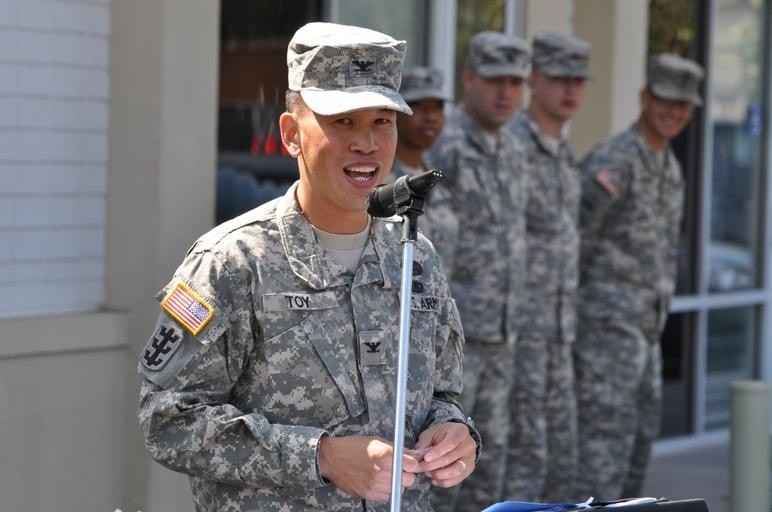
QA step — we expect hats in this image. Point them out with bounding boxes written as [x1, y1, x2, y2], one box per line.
[399, 66, 449, 102]
[468, 30, 594, 77]
[647, 52, 706, 106]
[286, 22, 413, 115]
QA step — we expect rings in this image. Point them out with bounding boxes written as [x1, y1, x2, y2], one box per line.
[455, 459, 467, 470]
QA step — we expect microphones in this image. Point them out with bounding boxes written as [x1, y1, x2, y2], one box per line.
[363, 168, 446, 218]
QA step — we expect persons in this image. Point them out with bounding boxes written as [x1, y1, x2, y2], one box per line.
[135, 19, 482, 512]
[428, 30, 534, 512]
[385, 63, 463, 285]
[576, 51, 708, 503]
[499, 29, 593, 505]
